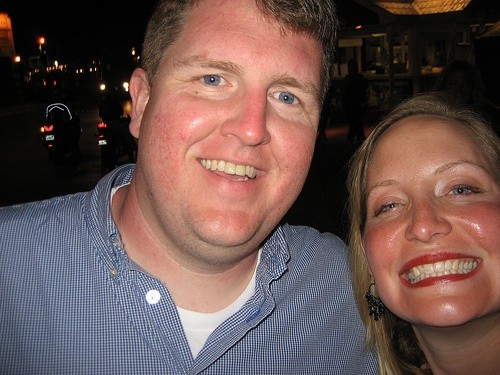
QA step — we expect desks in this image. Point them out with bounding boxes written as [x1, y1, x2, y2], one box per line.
[367, 68, 444, 103]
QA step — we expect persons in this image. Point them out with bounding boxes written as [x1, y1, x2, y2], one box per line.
[0, 0, 383, 375]
[315, 58, 473, 146]
[345, 92, 500, 373]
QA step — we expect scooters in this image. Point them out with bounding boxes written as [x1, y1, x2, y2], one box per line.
[97, 114, 137, 167]
[36, 108, 82, 162]
